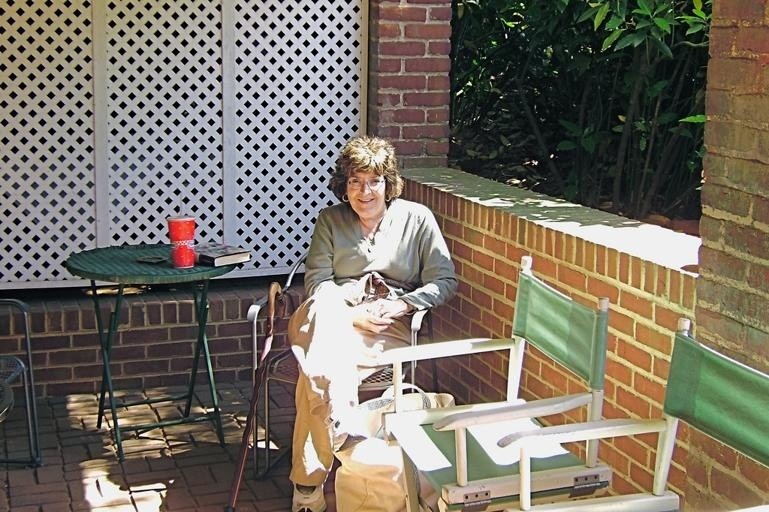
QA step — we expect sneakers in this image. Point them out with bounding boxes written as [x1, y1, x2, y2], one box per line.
[291, 483, 327, 512]
[323, 406, 370, 452]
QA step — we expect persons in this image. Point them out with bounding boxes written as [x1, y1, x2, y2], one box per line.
[287, 134, 459, 511]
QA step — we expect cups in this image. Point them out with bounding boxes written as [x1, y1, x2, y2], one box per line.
[166, 215, 197, 270]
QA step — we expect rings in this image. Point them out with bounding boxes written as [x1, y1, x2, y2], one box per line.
[381, 312, 385, 315]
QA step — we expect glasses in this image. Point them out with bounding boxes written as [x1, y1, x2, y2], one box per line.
[345, 177, 386, 191]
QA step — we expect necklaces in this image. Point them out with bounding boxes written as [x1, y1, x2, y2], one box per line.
[359, 217, 383, 240]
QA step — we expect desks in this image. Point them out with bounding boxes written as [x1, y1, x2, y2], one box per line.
[65, 243, 240, 463]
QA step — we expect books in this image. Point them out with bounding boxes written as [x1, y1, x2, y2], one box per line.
[194, 241, 252, 268]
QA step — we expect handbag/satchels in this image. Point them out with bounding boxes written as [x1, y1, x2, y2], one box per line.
[335, 382, 455, 512]
